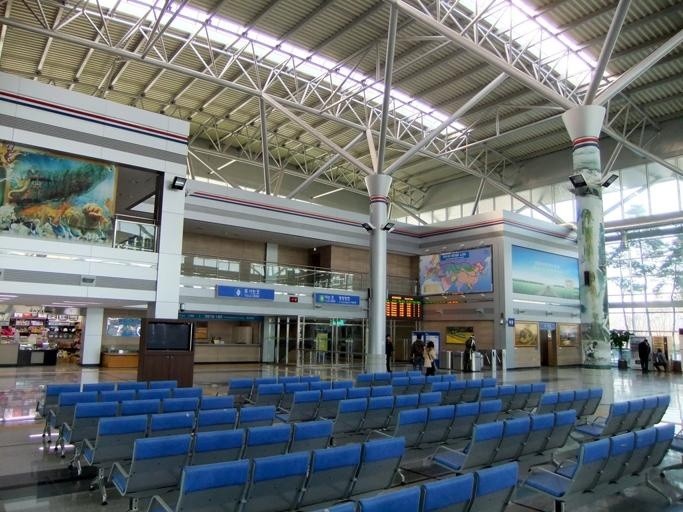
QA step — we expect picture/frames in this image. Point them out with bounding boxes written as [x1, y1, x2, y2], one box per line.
[558, 324, 578, 347]
[511, 244, 581, 301]
[3, 145, 118, 246]
[514, 322, 538, 347]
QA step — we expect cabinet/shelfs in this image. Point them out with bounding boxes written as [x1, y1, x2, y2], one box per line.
[232, 326, 253, 344]
[0, 317, 82, 363]
[136, 318, 195, 388]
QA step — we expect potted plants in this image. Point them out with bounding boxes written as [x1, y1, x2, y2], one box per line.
[609, 329, 629, 369]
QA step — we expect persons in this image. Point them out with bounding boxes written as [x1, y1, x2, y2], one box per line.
[386, 335, 393, 372]
[653, 348, 668, 373]
[638, 340, 650, 373]
[411, 334, 425, 370]
[422, 341, 437, 375]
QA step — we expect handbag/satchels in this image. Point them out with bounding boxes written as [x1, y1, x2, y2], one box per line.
[431, 361, 437, 372]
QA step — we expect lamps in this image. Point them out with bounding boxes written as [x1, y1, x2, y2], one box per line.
[361, 221, 376, 232]
[599, 172, 619, 188]
[568, 172, 587, 190]
[171, 176, 188, 191]
[381, 219, 396, 231]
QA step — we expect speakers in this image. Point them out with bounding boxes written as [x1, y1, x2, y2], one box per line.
[584, 270, 589, 285]
